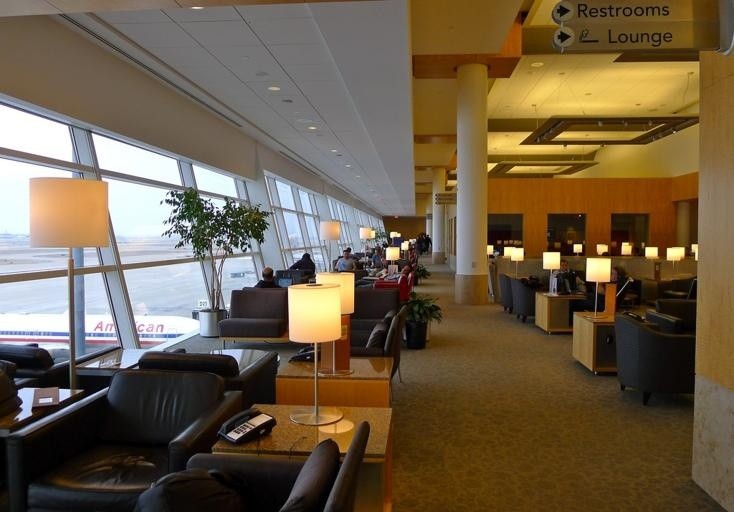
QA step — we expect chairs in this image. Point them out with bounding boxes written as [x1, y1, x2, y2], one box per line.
[496, 273, 697, 407]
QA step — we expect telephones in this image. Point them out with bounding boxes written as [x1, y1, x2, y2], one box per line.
[622, 311, 646, 323]
[288, 345, 320, 363]
[216, 408, 276, 444]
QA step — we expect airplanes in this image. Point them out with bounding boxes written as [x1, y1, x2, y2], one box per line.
[0, 307, 201, 347]
[292, 253, 323, 265]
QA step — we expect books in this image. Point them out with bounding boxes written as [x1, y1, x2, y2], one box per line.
[32, 387, 59, 411]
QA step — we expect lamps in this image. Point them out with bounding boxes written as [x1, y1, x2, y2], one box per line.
[486, 239, 699, 319]
[359, 226, 418, 277]
[319, 221, 341, 274]
[28, 176, 112, 392]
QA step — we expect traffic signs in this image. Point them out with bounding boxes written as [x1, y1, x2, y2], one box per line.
[553, 24, 719, 52]
[551, 1, 720, 24]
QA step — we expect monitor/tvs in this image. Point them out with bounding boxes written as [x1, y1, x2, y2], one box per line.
[557, 273, 576, 295]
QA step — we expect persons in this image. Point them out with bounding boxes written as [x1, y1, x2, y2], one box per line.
[254, 267, 282, 288]
[384, 265, 413, 285]
[376, 255, 402, 278]
[347, 248, 360, 261]
[414, 231, 432, 255]
[361, 244, 372, 253]
[370, 246, 385, 268]
[556, 260, 579, 294]
[290, 253, 315, 273]
[334, 250, 357, 272]
[588, 266, 627, 311]
[381, 241, 388, 248]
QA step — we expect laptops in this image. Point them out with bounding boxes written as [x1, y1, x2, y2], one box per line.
[277, 277, 295, 288]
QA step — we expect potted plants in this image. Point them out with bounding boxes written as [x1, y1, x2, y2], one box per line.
[159, 186, 275, 339]
[399, 292, 444, 350]
[414, 264, 432, 285]
